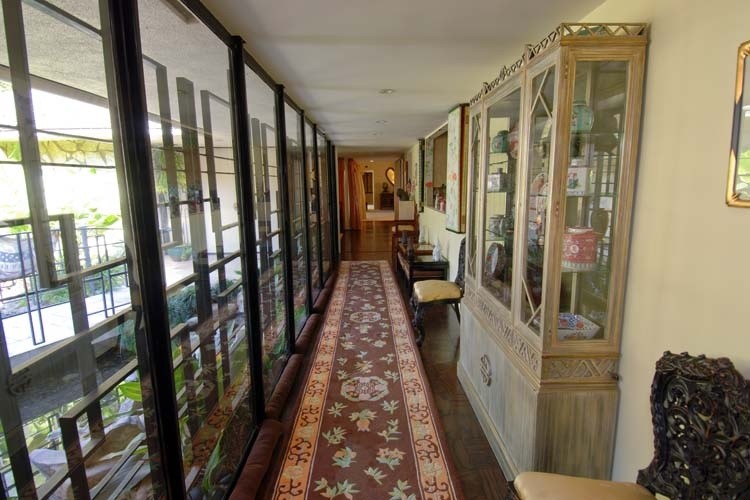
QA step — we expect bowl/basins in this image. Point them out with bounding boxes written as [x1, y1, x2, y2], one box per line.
[533, 313, 600, 340]
[486, 243, 507, 280]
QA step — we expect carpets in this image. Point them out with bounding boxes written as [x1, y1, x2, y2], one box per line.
[360, 210, 395, 221]
[270, 260, 465, 500]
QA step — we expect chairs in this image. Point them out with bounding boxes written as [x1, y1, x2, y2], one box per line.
[505, 350, 750, 500]
[409, 238, 466, 348]
[391, 213, 420, 255]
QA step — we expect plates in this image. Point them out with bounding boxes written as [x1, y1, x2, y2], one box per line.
[508, 132, 518, 160]
[531, 173, 549, 208]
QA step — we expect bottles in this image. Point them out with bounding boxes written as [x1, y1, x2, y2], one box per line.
[408, 236, 415, 258]
[418, 227, 425, 242]
[402, 231, 407, 244]
[435, 192, 445, 212]
[425, 182, 433, 206]
[567, 159, 593, 193]
[433, 240, 440, 261]
[562, 225, 597, 269]
[488, 168, 507, 190]
[491, 130, 509, 153]
[570, 100, 595, 133]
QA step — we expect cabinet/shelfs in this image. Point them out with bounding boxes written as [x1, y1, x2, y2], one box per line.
[380, 193, 394, 210]
[456, 22, 649, 482]
[362, 173, 372, 192]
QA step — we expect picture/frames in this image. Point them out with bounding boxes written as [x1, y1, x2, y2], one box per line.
[725, 41, 750, 209]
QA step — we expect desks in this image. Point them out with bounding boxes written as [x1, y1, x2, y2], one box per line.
[397, 230, 449, 300]
